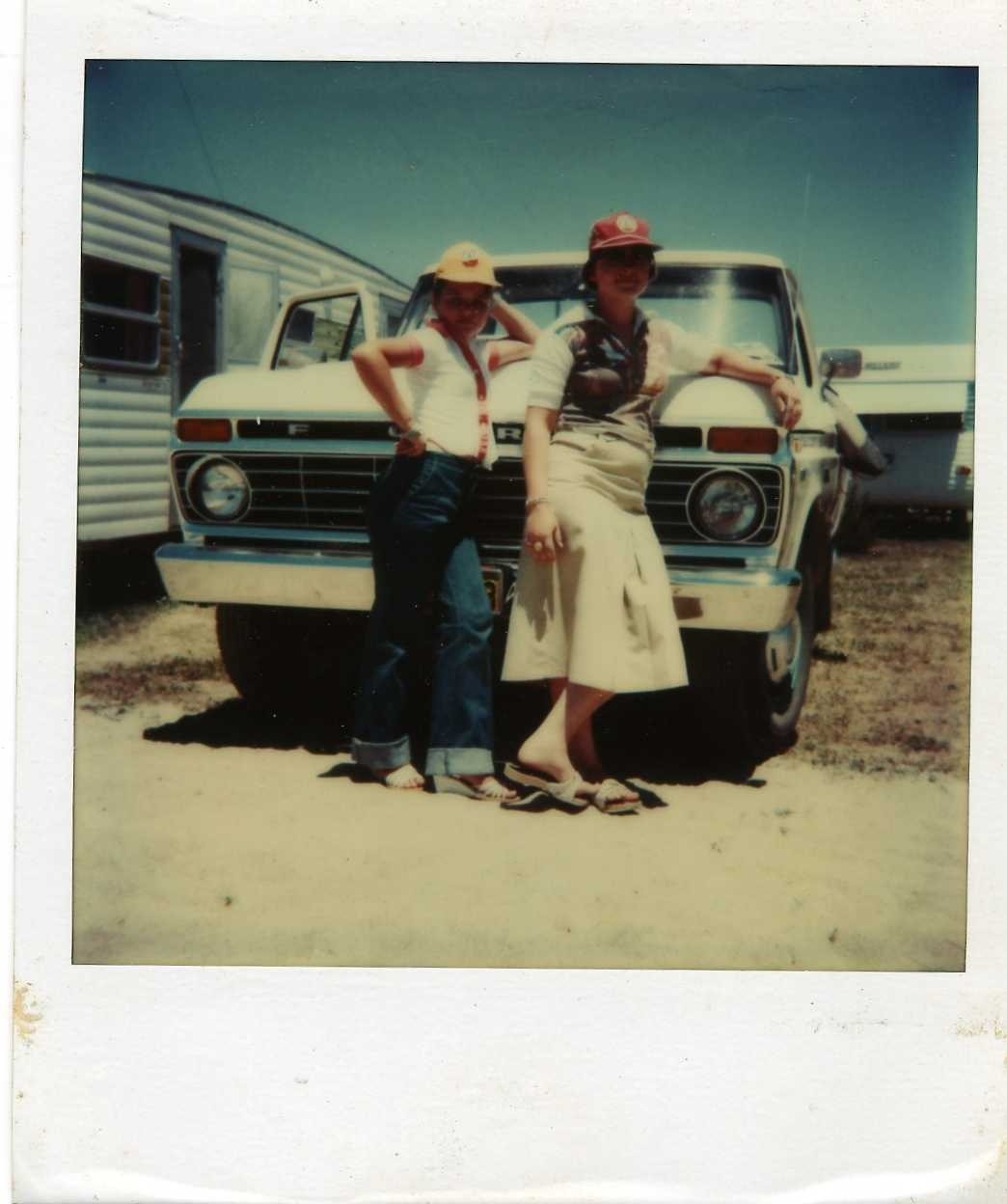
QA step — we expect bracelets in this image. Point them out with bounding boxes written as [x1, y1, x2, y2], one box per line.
[525, 495, 551, 508]
[772, 374, 795, 385]
[389, 422, 423, 438]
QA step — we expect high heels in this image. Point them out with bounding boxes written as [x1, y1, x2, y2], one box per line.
[374, 762, 425, 789]
[433, 773, 521, 803]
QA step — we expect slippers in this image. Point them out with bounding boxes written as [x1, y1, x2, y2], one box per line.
[509, 766, 589, 808]
[590, 780, 643, 812]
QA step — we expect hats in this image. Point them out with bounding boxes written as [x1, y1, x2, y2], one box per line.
[589, 210, 666, 251]
[436, 241, 503, 286]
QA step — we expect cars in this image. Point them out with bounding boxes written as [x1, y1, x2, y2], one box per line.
[154, 250, 863, 755]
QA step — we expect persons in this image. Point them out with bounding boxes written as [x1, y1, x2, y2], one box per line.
[350, 241, 542, 803]
[501, 211, 805, 814]
